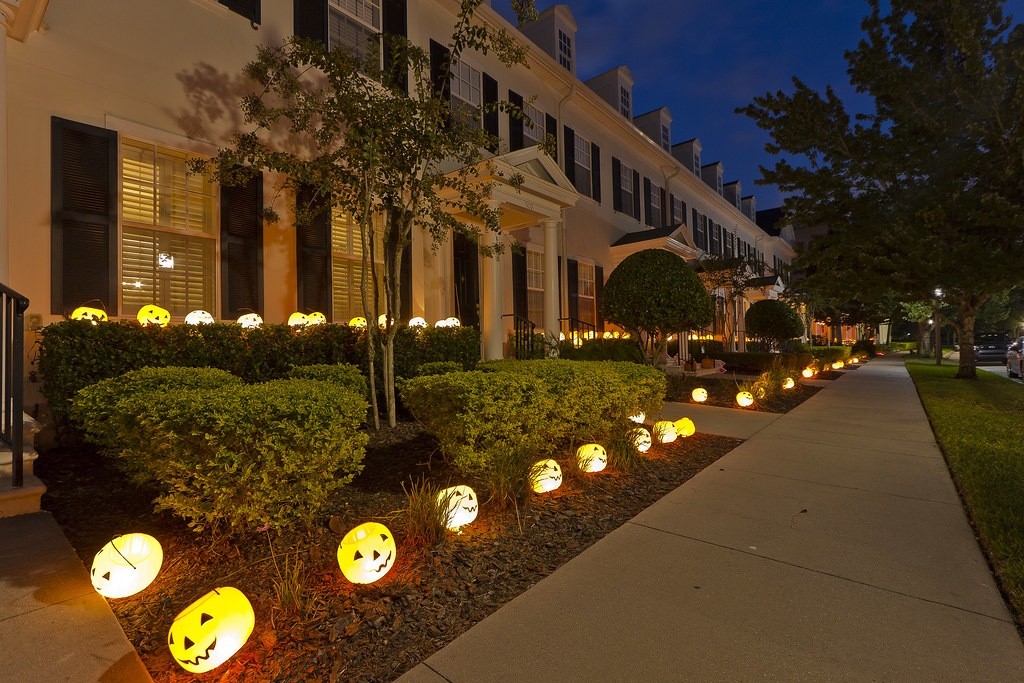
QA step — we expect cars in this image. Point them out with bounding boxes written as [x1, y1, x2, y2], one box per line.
[1005, 336, 1024, 383]
[973, 330, 1013, 366]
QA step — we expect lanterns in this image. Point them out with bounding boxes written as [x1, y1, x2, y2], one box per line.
[542, 331, 714, 349]
[69, 304, 327, 330]
[349, 313, 395, 334]
[336, 522, 397, 584]
[576, 443, 607, 472]
[434, 485, 479, 532]
[91, 532, 164, 598]
[802, 352, 884, 378]
[624, 409, 695, 453]
[735, 391, 753, 407]
[408, 316, 460, 335]
[692, 388, 707, 402]
[526, 458, 563, 493]
[167, 586, 256, 674]
[751, 372, 795, 399]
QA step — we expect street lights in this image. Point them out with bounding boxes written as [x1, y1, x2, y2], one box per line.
[928, 287, 944, 365]
